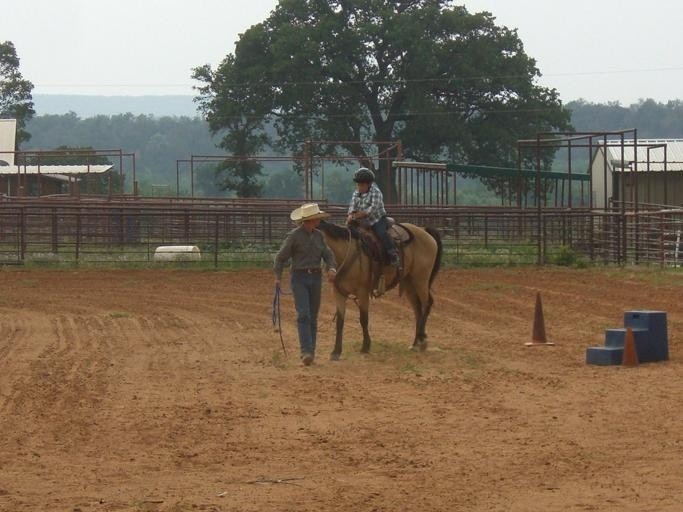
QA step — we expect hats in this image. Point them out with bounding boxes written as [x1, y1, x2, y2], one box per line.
[290, 202, 330, 221]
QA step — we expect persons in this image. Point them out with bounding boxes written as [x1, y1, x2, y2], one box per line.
[347, 168, 400, 266]
[273, 203, 337, 365]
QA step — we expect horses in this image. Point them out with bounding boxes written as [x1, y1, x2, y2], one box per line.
[297, 223, 442, 360]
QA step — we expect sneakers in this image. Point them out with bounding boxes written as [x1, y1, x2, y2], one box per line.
[301, 353, 315, 366]
[387, 252, 400, 264]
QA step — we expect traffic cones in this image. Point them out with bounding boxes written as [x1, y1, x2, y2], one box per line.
[523, 289, 558, 347]
[618, 326, 642, 369]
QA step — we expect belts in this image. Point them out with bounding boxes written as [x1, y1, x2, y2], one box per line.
[294, 268, 321, 272]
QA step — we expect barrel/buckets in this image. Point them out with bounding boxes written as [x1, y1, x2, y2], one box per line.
[154, 246, 201, 264]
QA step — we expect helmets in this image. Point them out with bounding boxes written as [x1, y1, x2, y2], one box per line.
[353, 168, 375, 181]
